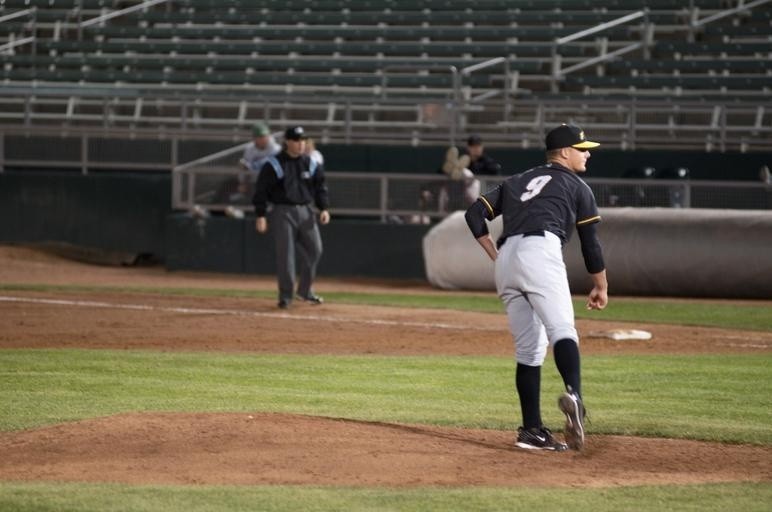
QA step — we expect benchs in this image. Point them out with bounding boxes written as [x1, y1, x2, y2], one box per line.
[0, 3, 771, 151]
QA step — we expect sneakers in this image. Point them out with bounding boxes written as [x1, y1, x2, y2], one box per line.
[278, 298, 292, 309]
[515, 425, 569, 452]
[558, 390, 587, 453]
[295, 292, 325, 305]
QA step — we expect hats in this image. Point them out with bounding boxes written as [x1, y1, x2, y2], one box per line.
[251, 122, 270, 138]
[284, 125, 311, 141]
[466, 134, 484, 146]
[544, 122, 601, 152]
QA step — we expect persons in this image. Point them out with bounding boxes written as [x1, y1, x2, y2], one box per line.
[415, 133, 501, 210]
[251, 125, 335, 311]
[463, 122, 610, 455]
[192, 121, 283, 223]
[301, 130, 326, 167]
